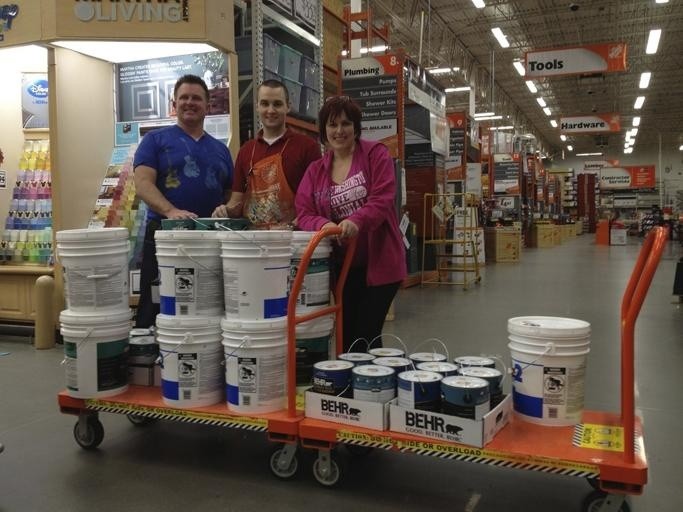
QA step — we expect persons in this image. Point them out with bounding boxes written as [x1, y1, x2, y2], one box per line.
[293, 95, 408, 353]
[210, 79, 323, 230]
[132, 74, 235, 329]
[214, 76, 229, 88]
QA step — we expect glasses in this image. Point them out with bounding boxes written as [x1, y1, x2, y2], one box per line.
[324, 95, 350, 104]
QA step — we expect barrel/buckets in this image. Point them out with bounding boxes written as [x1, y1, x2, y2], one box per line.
[53, 216, 508, 427]
[506, 315, 590, 426]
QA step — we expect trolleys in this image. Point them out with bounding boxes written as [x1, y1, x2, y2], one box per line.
[297, 226, 669, 512]
[56, 223, 359, 482]
